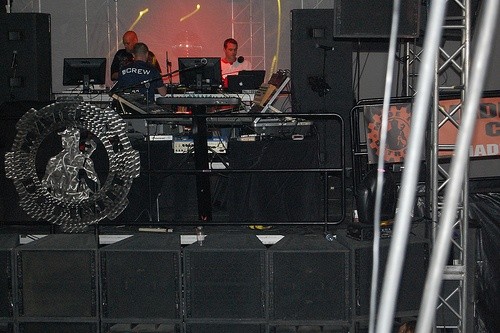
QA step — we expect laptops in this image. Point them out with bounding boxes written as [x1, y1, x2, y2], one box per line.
[228, 74, 259, 94]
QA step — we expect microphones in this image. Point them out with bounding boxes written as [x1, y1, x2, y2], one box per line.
[315, 44, 335, 51]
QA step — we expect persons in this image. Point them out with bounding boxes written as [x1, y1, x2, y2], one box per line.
[221, 38, 252, 86]
[117, 44, 167, 101]
[111, 31, 162, 79]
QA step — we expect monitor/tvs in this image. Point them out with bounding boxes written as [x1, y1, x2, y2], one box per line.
[178, 57, 222, 93]
[63, 58, 106, 94]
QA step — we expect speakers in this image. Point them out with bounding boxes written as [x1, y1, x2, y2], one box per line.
[0, 12, 52, 102]
[290, 9, 355, 170]
[333, 0, 421, 38]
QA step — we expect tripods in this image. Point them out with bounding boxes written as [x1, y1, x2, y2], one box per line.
[113, 63, 204, 229]
